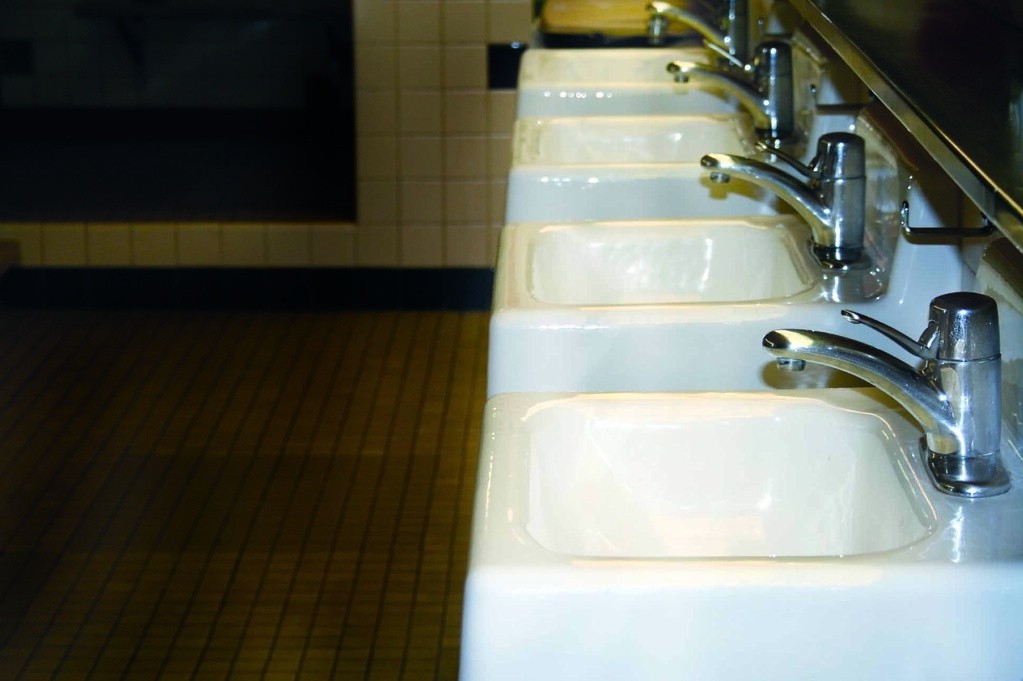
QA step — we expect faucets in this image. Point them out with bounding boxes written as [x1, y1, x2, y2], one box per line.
[760, 290, 1013, 499]
[700, 130, 873, 272]
[665, 39, 807, 161]
[644, 0, 751, 71]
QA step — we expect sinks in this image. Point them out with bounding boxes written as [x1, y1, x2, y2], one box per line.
[516, 46, 726, 90]
[474, 389, 955, 574]
[496, 215, 827, 311]
[510, 112, 753, 176]
